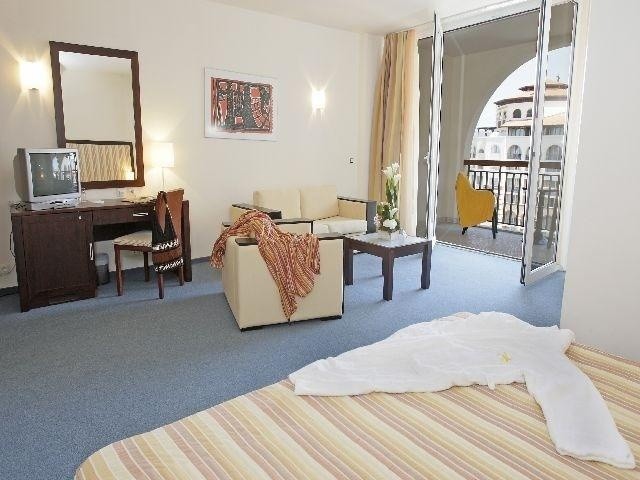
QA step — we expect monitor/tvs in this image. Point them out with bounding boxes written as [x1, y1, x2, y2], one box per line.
[13, 147, 82, 211]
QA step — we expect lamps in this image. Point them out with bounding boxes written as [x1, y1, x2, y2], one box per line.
[20, 56, 45, 96]
[145, 140, 178, 187]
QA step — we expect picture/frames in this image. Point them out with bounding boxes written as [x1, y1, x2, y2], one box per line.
[203, 66, 279, 142]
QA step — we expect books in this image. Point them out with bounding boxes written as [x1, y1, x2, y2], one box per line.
[121, 193, 156, 203]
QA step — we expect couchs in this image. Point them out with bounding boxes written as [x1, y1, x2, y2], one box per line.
[231, 183, 378, 255]
[208, 216, 345, 332]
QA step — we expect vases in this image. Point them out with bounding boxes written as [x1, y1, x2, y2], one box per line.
[378, 228, 400, 240]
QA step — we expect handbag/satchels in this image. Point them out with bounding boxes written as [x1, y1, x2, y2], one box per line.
[151, 192, 183, 274]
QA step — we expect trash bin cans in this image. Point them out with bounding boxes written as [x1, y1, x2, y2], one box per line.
[93, 252, 110, 285]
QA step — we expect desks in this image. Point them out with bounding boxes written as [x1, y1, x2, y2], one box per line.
[9, 197, 192, 313]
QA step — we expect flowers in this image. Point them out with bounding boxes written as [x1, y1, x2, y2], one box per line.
[373, 163, 405, 234]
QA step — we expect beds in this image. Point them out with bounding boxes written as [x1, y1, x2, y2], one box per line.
[71, 311, 640, 480]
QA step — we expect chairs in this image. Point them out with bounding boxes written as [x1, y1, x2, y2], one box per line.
[455, 171, 499, 239]
[113, 188, 185, 298]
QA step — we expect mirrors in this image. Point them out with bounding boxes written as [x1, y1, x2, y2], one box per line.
[50, 41, 146, 189]
